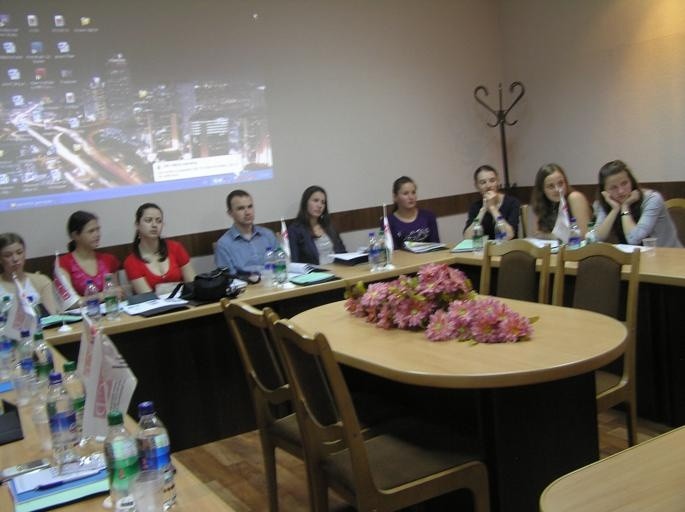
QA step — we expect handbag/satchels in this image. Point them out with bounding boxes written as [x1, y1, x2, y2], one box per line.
[182, 265, 234, 302]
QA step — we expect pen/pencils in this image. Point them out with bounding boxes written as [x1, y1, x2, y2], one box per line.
[37, 470, 101, 491]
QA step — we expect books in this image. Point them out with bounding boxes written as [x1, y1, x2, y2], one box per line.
[325, 250, 370, 267]
[451, 237, 490, 254]
[5, 453, 126, 512]
[398, 239, 449, 254]
[119, 297, 189, 316]
[228, 278, 248, 291]
[282, 261, 317, 275]
[288, 270, 337, 287]
[37, 314, 80, 329]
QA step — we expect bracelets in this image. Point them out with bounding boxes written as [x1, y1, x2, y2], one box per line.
[619, 211, 633, 216]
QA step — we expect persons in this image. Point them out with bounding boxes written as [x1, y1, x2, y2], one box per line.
[388, 176, 440, 250]
[287, 184, 347, 264]
[211, 189, 285, 275]
[1, 232, 64, 316]
[461, 164, 524, 240]
[524, 162, 593, 241]
[587, 159, 684, 248]
[123, 202, 196, 294]
[14, 108, 156, 190]
[54, 210, 121, 310]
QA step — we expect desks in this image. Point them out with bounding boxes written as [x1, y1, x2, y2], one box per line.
[458, 237, 684, 426]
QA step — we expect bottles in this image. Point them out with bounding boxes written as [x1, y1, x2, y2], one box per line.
[103, 274, 119, 322]
[133, 400, 177, 510]
[0, 292, 91, 472]
[83, 279, 101, 324]
[472, 218, 483, 254]
[494, 215, 507, 246]
[260, 245, 288, 290]
[103, 410, 145, 512]
[585, 222, 597, 245]
[368, 230, 388, 274]
[568, 218, 581, 250]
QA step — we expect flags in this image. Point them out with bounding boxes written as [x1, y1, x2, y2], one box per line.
[72, 310, 137, 445]
[279, 221, 291, 256]
[549, 193, 573, 241]
[18, 277, 40, 305]
[4, 281, 36, 341]
[383, 218, 395, 257]
[49, 265, 80, 312]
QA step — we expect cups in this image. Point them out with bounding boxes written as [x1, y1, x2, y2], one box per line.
[642, 237, 658, 256]
[128, 468, 163, 512]
